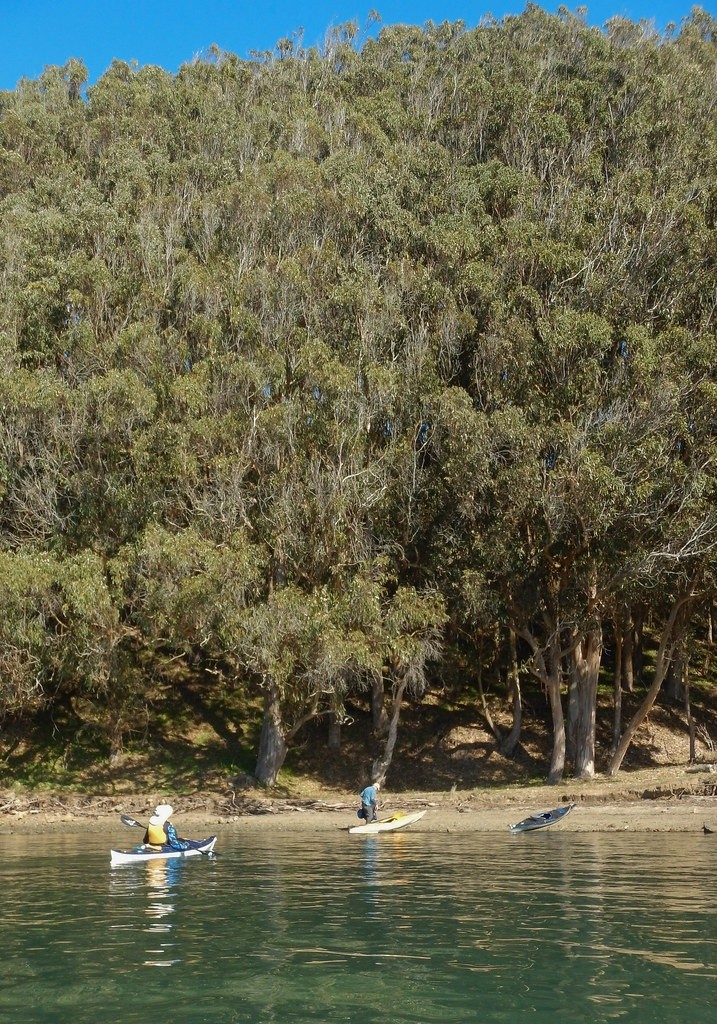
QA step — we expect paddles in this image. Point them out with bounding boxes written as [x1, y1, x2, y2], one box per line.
[121, 814, 221, 855]
[370, 812, 403, 823]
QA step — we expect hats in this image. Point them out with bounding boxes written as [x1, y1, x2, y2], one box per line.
[149, 804, 173, 826]
[373, 782, 381, 791]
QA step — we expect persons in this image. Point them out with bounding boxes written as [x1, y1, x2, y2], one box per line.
[360, 782, 381, 824]
[143, 804, 190, 850]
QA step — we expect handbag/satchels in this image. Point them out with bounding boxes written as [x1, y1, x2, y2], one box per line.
[357, 809, 378, 820]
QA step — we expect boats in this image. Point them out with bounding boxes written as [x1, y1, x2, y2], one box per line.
[510, 804, 577, 834]
[349, 809, 428, 834]
[111, 835, 217, 865]
